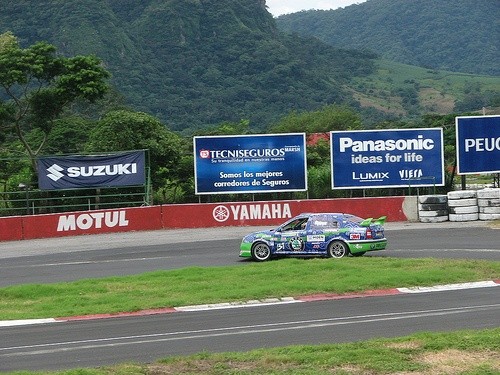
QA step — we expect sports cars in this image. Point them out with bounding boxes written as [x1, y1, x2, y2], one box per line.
[239, 213, 387, 262]
